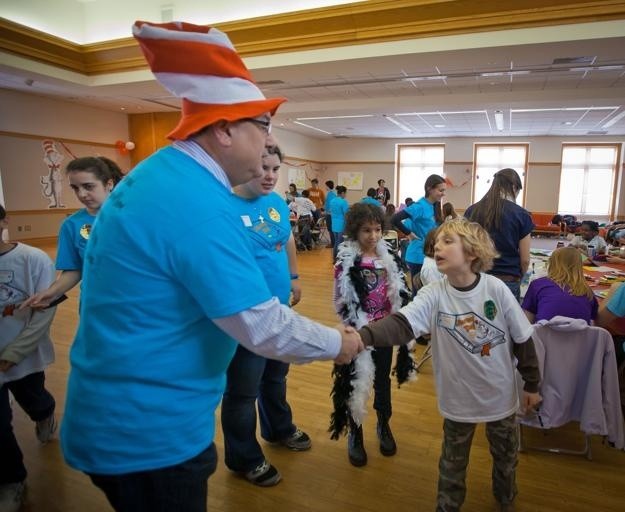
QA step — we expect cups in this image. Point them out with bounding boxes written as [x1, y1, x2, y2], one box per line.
[586, 248, 597, 256]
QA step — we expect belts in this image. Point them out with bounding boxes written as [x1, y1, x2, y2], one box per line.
[492, 272, 522, 283]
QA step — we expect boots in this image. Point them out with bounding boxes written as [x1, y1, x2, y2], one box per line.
[347, 412, 368, 467]
[376, 408, 397, 456]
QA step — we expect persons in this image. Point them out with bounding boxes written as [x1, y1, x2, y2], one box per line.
[344, 217, 541, 512]
[59, 111, 364, 512]
[327, 202, 416, 467]
[221, 145, 313, 487]
[18, 157, 127, 311]
[282, 174, 459, 346]
[594, 280, 625, 416]
[521, 247, 599, 326]
[0, 205, 59, 512]
[464, 168, 536, 304]
[567, 221, 608, 257]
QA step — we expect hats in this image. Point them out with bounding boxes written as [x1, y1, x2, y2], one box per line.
[132, 17, 289, 141]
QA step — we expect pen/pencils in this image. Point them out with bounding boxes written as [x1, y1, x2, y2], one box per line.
[537, 407, 544, 426]
[532, 263, 535, 275]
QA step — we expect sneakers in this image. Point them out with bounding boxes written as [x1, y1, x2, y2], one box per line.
[223, 456, 282, 487]
[0, 479, 28, 512]
[297, 243, 332, 251]
[261, 428, 312, 451]
[32, 414, 58, 443]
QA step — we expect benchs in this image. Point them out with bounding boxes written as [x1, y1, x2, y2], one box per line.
[561, 214, 610, 238]
[529, 212, 561, 239]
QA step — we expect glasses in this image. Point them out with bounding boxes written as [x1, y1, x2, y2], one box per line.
[235, 115, 272, 137]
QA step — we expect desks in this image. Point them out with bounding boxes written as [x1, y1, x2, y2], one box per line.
[516, 248, 625, 336]
[290, 218, 299, 233]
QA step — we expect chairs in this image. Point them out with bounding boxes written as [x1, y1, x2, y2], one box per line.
[292, 214, 310, 254]
[413, 271, 433, 373]
[309, 216, 329, 249]
[382, 230, 398, 254]
[516, 323, 609, 461]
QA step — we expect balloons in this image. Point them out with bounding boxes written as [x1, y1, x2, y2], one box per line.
[116, 140, 125, 149]
[125, 142, 135, 151]
[120, 148, 128, 156]
